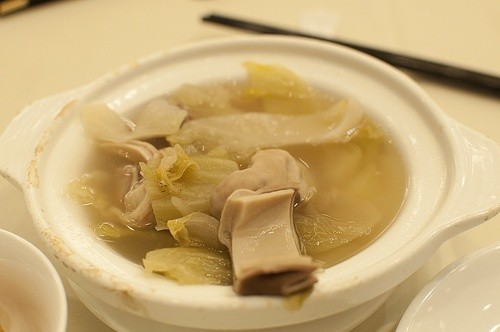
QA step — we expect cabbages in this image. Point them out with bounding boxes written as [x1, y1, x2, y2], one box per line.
[66, 59, 387, 283]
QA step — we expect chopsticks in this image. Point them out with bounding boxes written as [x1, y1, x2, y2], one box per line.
[203, 15, 500, 94]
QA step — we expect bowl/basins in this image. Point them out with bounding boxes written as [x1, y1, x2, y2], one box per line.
[1, 34, 500, 330]
